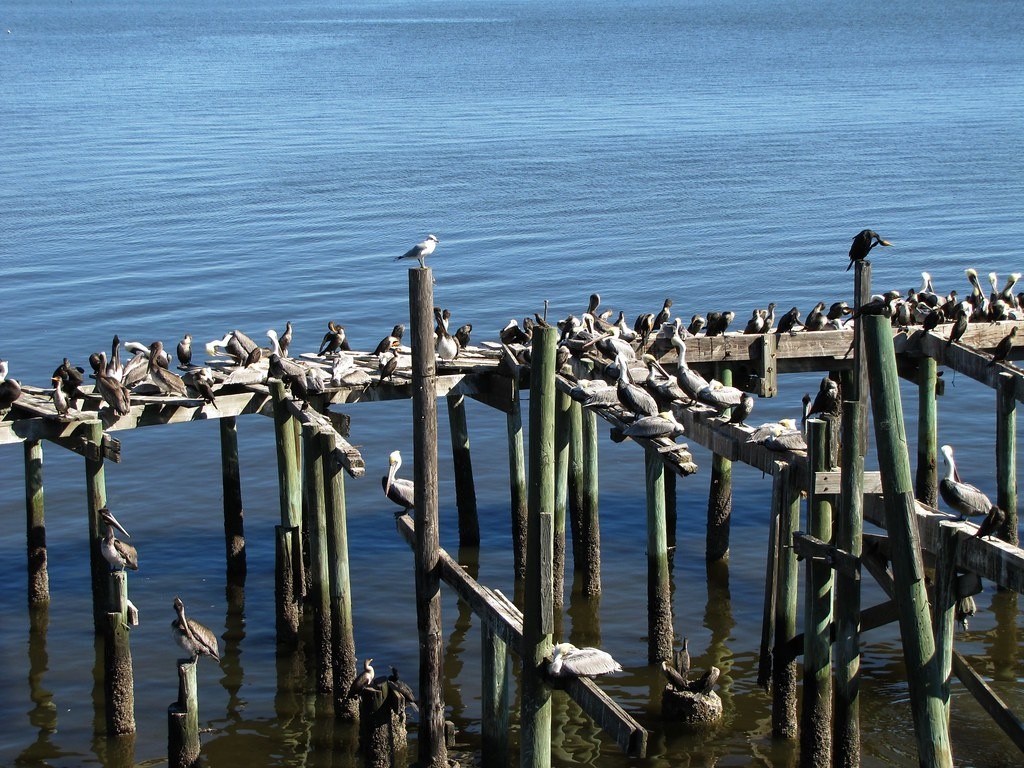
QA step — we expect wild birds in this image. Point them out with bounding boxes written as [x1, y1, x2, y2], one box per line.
[660, 634, 721, 695]
[845, 229, 895, 272]
[96, 505, 140, 572]
[168, 594, 224, 663]
[546, 642, 624, 681]
[392, 234, 440, 269]
[344, 656, 420, 714]
[0, 267, 1024, 543]
